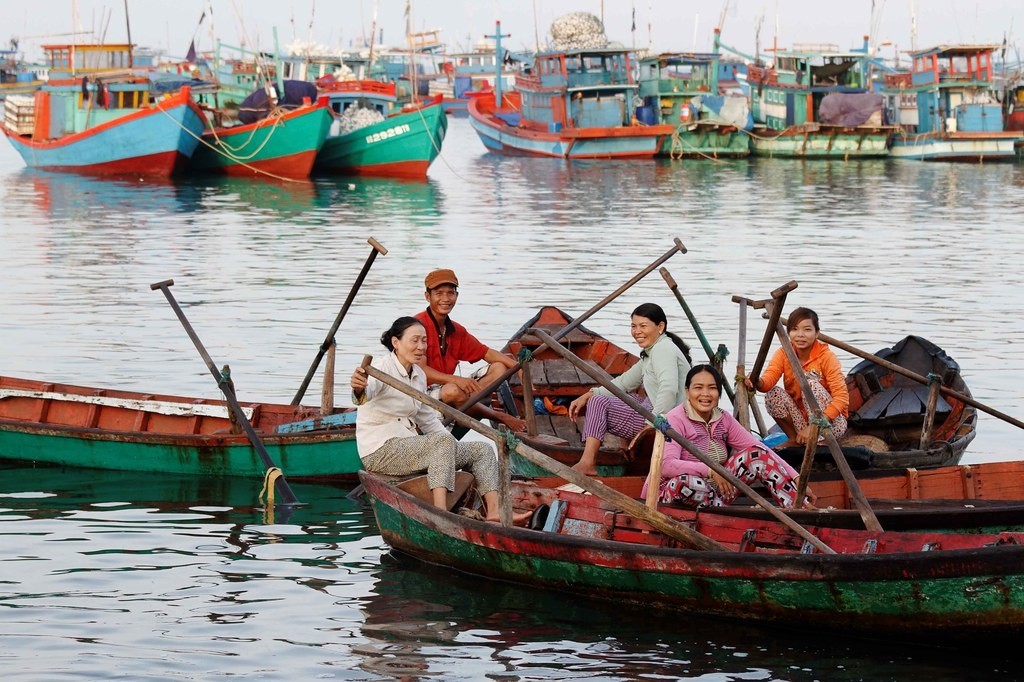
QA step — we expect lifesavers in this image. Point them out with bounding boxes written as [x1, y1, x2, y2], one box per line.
[680, 103, 690, 122]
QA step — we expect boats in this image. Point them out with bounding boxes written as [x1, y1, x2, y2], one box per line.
[0, 376, 366, 479]
[760, 336, 978, 478]
[159, 0, 488, 113]
[465, 1, 1023, 166]
[130, 67, 335, 181]
[452, 306, 656, 480]
[0, 0, 209, 180]
[359, 461, 1023, 640]
[276, 54, 446, 179]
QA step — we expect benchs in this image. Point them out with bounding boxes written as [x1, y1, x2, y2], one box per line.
[507, 359, 614, 396]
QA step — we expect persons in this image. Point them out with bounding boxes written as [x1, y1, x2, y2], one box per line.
[979, 91, 1001, 103]
[569, 303, 691, 476]
[350, 317, 533, 523]
[744, 306, 850, 449]
[631, 114, 640, 126]
[659, 364, 837, 512]
[412, 268, 536, 433]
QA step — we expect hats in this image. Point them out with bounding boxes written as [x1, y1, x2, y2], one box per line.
[424, 269, 458, 287]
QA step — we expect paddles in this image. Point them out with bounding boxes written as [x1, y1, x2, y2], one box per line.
[730, 293, 751, 434]
[526, 328, 836, 555]
[345, 237, 690, 500]
[658, 267, 734, 403]
[290, 238, 389, 404]
[150, 278, 298, 504]
[355, 353, 721, 552]
[750, 280, 798, 387]
[753, 298, 883, 530]
[762, 312, 1024, 429]
